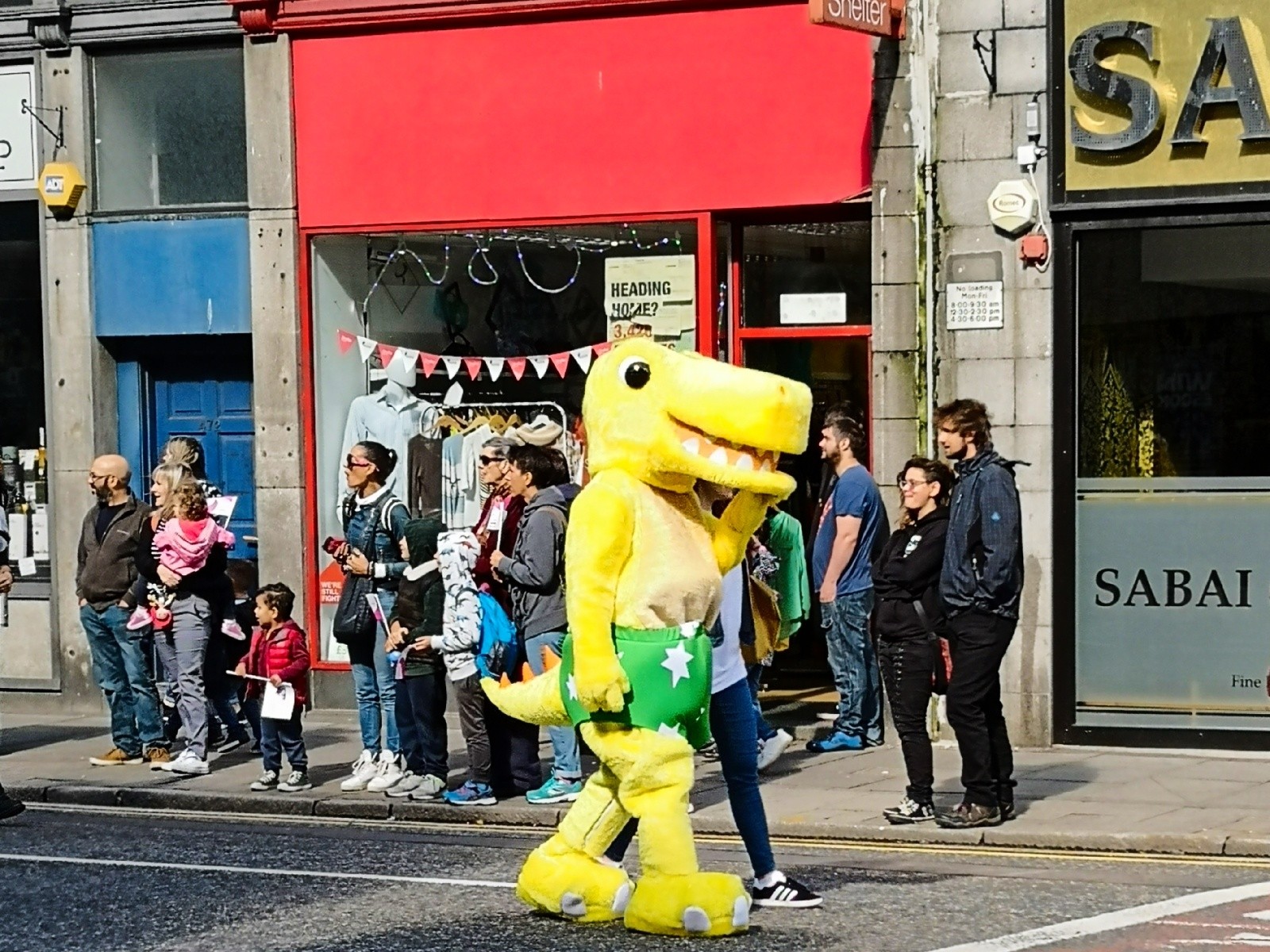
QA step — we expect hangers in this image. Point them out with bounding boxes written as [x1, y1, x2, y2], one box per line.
[420, 400, 554, 439]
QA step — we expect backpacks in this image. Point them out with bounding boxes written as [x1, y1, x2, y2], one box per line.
[455, 587, 516, 681]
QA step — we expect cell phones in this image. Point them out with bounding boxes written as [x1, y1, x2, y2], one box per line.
[327, 540, 347, 553]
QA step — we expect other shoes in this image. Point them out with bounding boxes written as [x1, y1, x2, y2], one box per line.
[697, 740, 720, 762]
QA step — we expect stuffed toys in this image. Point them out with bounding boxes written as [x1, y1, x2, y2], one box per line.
[479, 337, 814, 937]
[147, 581, 177, 627]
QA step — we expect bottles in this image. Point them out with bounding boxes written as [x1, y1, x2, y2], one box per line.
[13, 481, 25, 506]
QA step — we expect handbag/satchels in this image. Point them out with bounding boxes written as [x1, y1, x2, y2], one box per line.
[332, 538, 377, 644]
[927, 633, 953, 696]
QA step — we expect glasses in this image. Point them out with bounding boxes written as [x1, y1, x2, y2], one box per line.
[89, 471, 109, 480]
[479, 455, 505, 466]
[899, 480, 929, 490]
[347, 454, 380, 472]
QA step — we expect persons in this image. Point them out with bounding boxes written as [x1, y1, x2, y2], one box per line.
[75, 453, 169, 771]
[334, 442, 413, 792]
[134, 436, 251, 774]
[126, 480, 246, 642]
[805, 412, 890, 752]
[336, 349, 441, 526]
[235, 581, 314, 793]
[0, 502, 26, 824]
[871, 457, 956, 825]
[709, 559, 826, 908]
[382, 437, 583, 806]
[933, 399, 1025, 830]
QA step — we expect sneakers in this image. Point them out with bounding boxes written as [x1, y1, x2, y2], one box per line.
[276, 770, 313, 793]
[126, 608, 153, 630]
[953, 801, 1017, 820]
[756, 728, 794, 770]
[221, 620, 246, 641]
[384, 770, 448, 800]
[882, 796, 935, 823]
[0, 789, 28, 826]
[934, 802, 1002, 828]
[341, 749, 406, 793]
[249, 771, 280, 791]
[442, 779, 498, 806]
[525, 767, 582, 804]
[89, 730, 263, 775]
[805, 730, 865, 754]
[749, 876, 824, 908]
